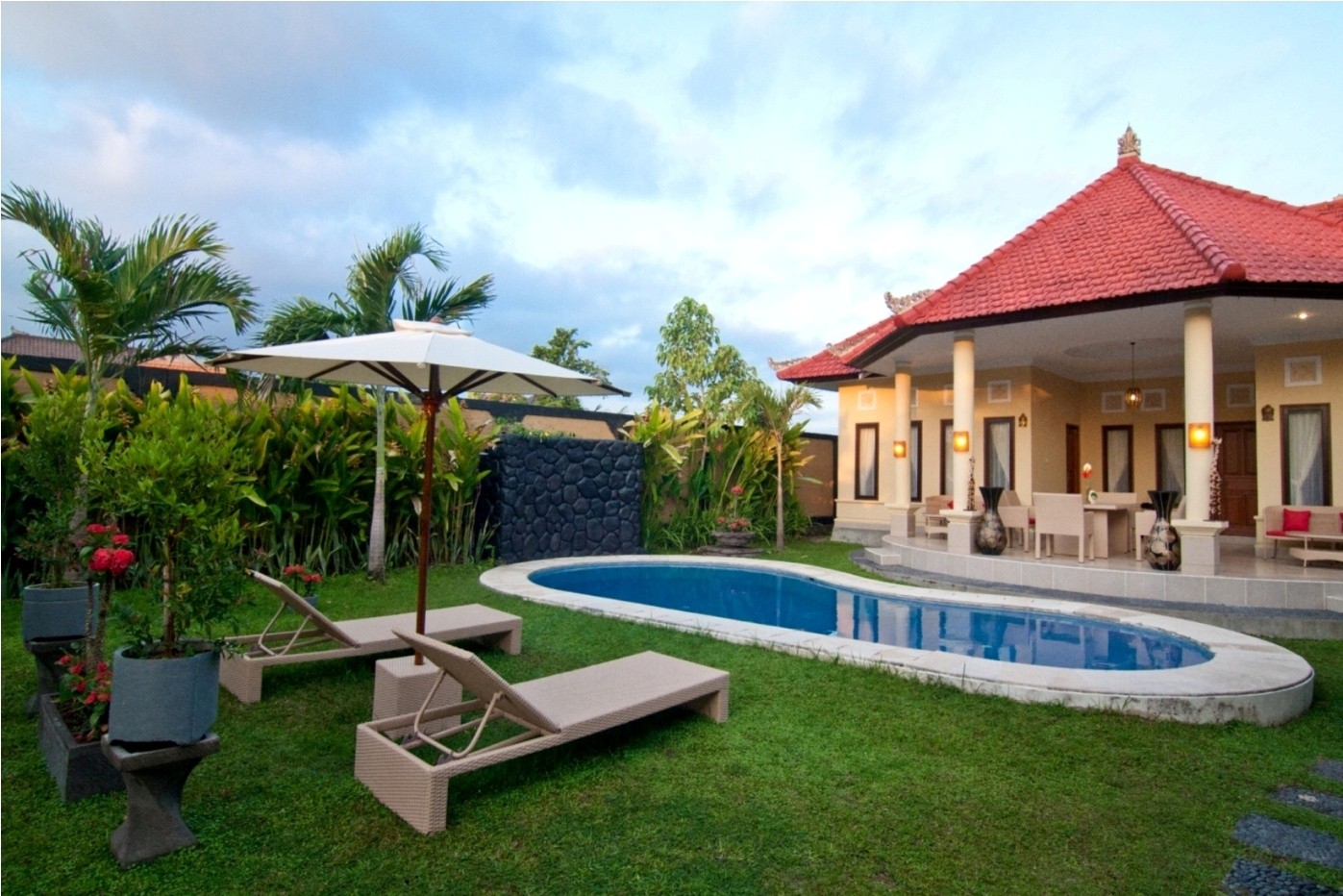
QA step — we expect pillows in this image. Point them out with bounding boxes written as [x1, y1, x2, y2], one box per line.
[1282, 507, 1311, 531]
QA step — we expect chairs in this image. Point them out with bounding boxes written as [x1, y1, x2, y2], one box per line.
[216, 567, 523, 706]
[355, 626, 731, 834]
[922, 489, 1187, 563]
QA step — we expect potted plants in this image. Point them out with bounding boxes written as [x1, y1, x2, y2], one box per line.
[1, 355, 133, 638]
[74, 370, 268, 745]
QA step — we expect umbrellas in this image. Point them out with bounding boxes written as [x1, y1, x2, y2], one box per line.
[204, 317, 631, 739]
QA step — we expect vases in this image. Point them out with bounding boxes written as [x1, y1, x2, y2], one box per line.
[976, 485, 1007, 557]
[287, 594, 319, 615]
[710, 530, 754, 546]
[1144, 489, 1182, 571]
[38, 690, 127, 803]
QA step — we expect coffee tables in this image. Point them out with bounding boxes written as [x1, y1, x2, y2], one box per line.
[1285, 531, 1343, 567]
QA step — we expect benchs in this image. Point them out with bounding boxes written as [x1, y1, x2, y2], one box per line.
[1264, 505, 1343, 561]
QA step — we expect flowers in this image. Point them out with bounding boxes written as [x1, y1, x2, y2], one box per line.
[56, 514, 135, 741]
[283, 563, 322, 596]
[718, 485, 750, 532]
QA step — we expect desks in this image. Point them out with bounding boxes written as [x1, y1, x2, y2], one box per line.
[1053, 503, 1132, 557]
[373, 654, 462, 740]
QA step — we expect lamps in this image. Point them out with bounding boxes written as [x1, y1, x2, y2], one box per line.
[1190, 423, 1212, 448]
[893, 441, 905, 457]
[1125, 341, 1143, 408]
[1018, 413, 1027, 427]
[1262, 405, 1274, 421]
[951, 431, 969, 452]
